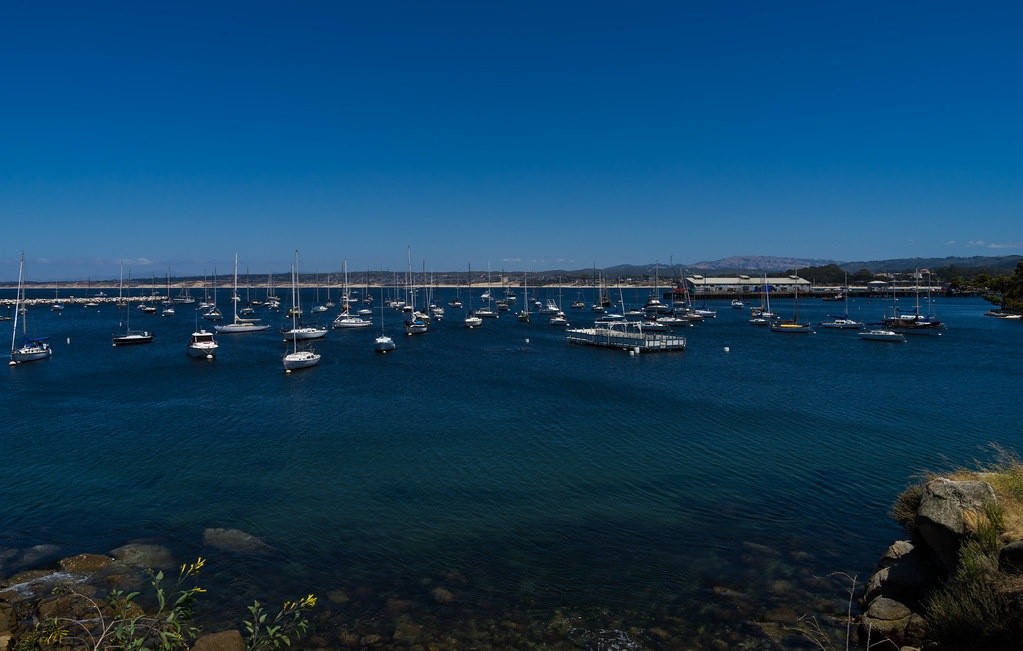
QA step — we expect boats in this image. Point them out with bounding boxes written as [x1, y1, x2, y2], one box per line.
[186, 308, 220, 359]
[17, 308, 29, 315]
[0, 315, 11, 321]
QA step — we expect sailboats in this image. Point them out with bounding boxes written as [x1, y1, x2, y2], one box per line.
[771, 267, 812, 334]
[10, 249, 53, 364]
[51, 278, 65, 311]
[281, 263, 322, 371]
[229, 265, 281, 323]
[866, 266, 941, 336]
[818, 272, 864, 329]
[111, 269, 154, 344]
[284, 260, 445, 342]
[114, 265, 223, 321]
[212, 253, 272, 333]
[855, 281, 907, 343]
[571, 254, 717, 331]
[730, 272, 744, 309]
[84, 275, 99, 308]
[375, 283, 396, 352]
[748, 272, 781, 326]
[446, 261, 567, 327]
